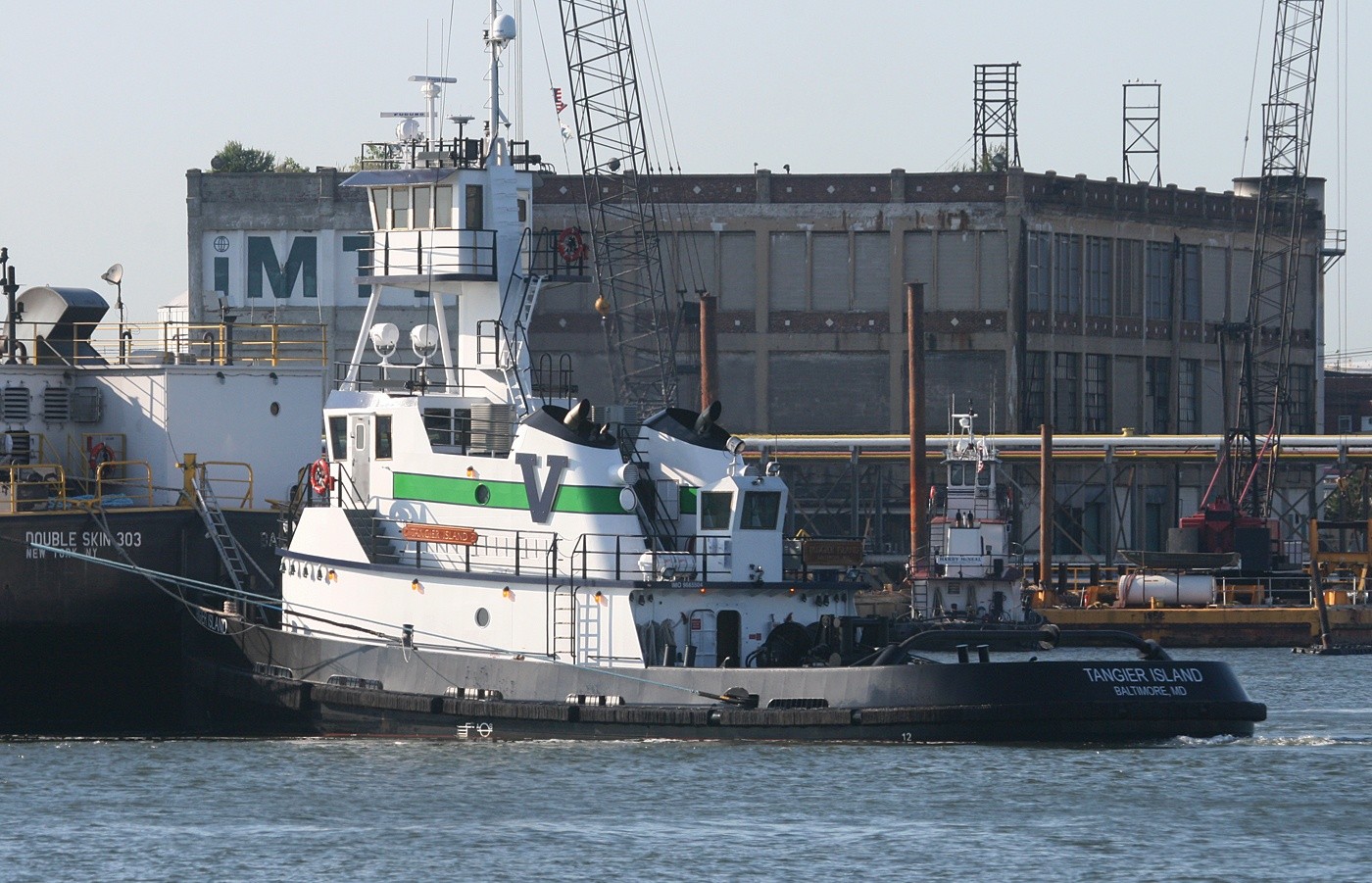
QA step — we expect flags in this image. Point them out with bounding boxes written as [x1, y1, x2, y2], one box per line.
[553, 87, 568, 113]
[561, 123, 574, 140]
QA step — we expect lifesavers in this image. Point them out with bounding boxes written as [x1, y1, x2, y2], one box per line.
[559, 226, 583, 261]
[90, 443, 116, 474]
[310, 460, 330, 493]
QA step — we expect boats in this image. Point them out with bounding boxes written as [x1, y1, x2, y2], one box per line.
[0, 242, 330, 719]
[173, 1, 1272, 755]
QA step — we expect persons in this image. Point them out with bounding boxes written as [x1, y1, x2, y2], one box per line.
[967, 510, 974, 528]
[955, 509, 962, 527]
